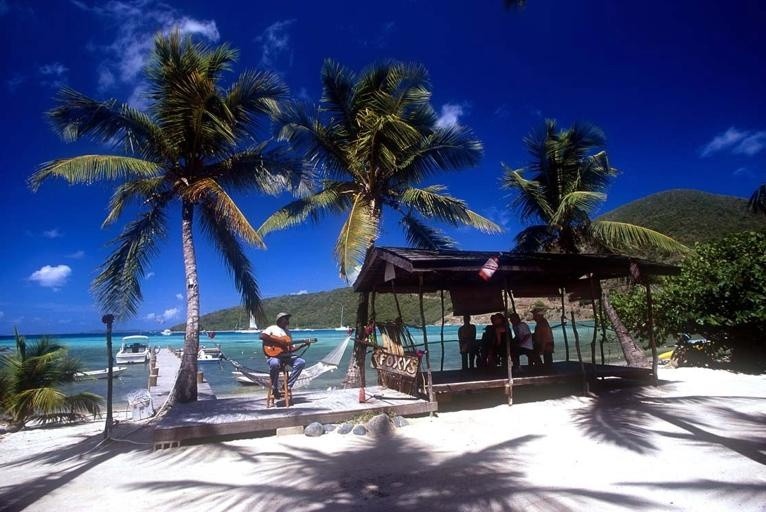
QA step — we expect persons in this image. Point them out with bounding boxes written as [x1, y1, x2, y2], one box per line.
[259, 311, 306, 400]
[478, 306, 554, 372]
[458, 314, 476, 370]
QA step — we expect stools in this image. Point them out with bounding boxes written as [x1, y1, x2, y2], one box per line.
[266, 362, 293, 407]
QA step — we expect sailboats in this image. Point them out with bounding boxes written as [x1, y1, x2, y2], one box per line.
[236, 310, 262, 333]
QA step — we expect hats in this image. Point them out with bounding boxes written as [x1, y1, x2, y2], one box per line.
[491, 313, 509, 325]
[530, 308, 546, 316]
[275, 312, 291, 323]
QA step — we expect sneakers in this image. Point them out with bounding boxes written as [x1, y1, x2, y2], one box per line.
[273, 390, 281, 399]
[287, 386, 290, 391]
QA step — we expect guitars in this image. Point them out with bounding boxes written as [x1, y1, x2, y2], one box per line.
[262, 336, 318, 357]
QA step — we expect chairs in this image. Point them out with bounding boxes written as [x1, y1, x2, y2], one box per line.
[125, 347, 144, 353]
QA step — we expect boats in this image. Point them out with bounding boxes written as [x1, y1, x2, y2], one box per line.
[161, 330, 172, 336]
[72, 366, 128, 381]
[116, 335, 151, 364]
[232, 370, 310, 385]
[177, 344, 223, 362]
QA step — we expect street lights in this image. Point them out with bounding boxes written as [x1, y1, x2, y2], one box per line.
[336, 302, 343, 327]
